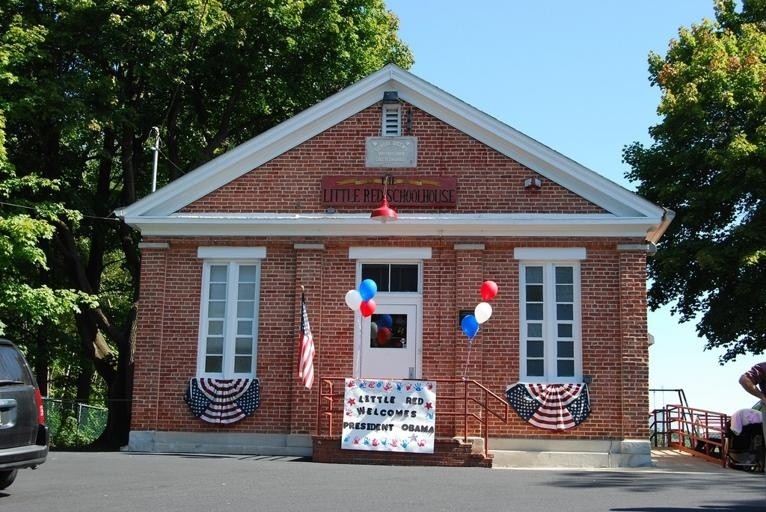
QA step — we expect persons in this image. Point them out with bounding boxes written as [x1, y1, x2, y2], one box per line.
[738, 361, 766, 446]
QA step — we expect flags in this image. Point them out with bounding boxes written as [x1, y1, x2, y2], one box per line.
[297, 291, 317, 390]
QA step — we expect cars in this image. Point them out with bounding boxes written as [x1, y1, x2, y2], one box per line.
[1, 338, 49, 490]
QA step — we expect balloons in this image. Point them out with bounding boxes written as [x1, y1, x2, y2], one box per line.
[461, 279, 499, 340]
[371, 314, 392, 345]
[344, 278, 377, 318]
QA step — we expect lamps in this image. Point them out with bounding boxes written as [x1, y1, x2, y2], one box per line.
[371, 175, 399, 224]
[525, 177, 542, 191]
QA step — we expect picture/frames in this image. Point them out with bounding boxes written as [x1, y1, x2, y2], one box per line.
[456, 308, 477, 329]
